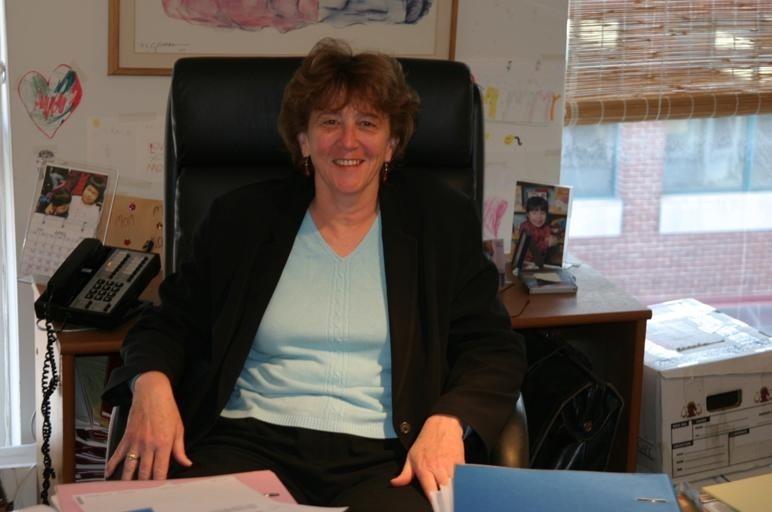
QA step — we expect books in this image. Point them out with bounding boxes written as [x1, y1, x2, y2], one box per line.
[75, 356, 118, 482]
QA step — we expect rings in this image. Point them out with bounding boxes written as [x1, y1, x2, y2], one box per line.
[127, 452, 141, 460]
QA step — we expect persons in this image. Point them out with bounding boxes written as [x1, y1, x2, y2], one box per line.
[520, 197, 558, 261]
[39, 173, 106, 227]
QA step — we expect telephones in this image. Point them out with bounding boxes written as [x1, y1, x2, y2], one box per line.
[34, 239, 162, 328]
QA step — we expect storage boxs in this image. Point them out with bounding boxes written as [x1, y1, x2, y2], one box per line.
[638, 297, 772, 490]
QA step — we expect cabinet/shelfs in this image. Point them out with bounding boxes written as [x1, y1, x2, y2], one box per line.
[33, 254, 652, 472]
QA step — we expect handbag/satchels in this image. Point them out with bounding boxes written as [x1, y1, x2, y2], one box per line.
[522, 327, 625, 471]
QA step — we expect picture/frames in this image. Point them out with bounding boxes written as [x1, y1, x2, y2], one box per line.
[18, 161, 120, 286]
[107, 0, 458, 77]
